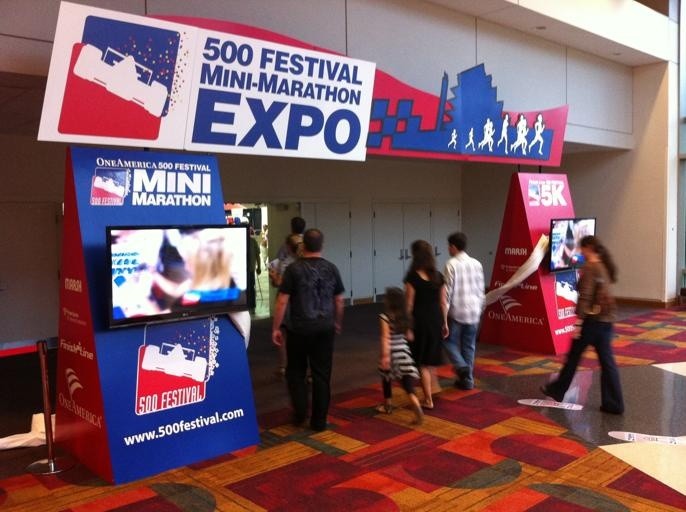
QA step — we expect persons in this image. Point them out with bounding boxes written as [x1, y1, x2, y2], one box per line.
[262, 224, 268, 271]
[374, 287, 424, 424]
[403, 240, 449, 410]
[152, 239, 230, 301]
[272, 229, 346, 431]
[564, 224, 591, 258]
[444, 232, 485, 388]
[268, 235, 304, 373]
[277, 217, 306, 261]
[540, 236, 626, 415]
[240, 223, 260, 315]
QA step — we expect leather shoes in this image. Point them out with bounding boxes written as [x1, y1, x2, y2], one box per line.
[459, 366, 470, 379]
[454, 379, 473, 389]
[599, 403, 625, 415]
[541, 386, 564, 402]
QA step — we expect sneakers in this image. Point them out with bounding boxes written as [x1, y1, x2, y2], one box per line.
[250, 306, 255, 314]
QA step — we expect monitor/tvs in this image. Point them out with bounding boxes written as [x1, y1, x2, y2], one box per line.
[549, 218, 597, 273]
[105, 224, 255, 331]
